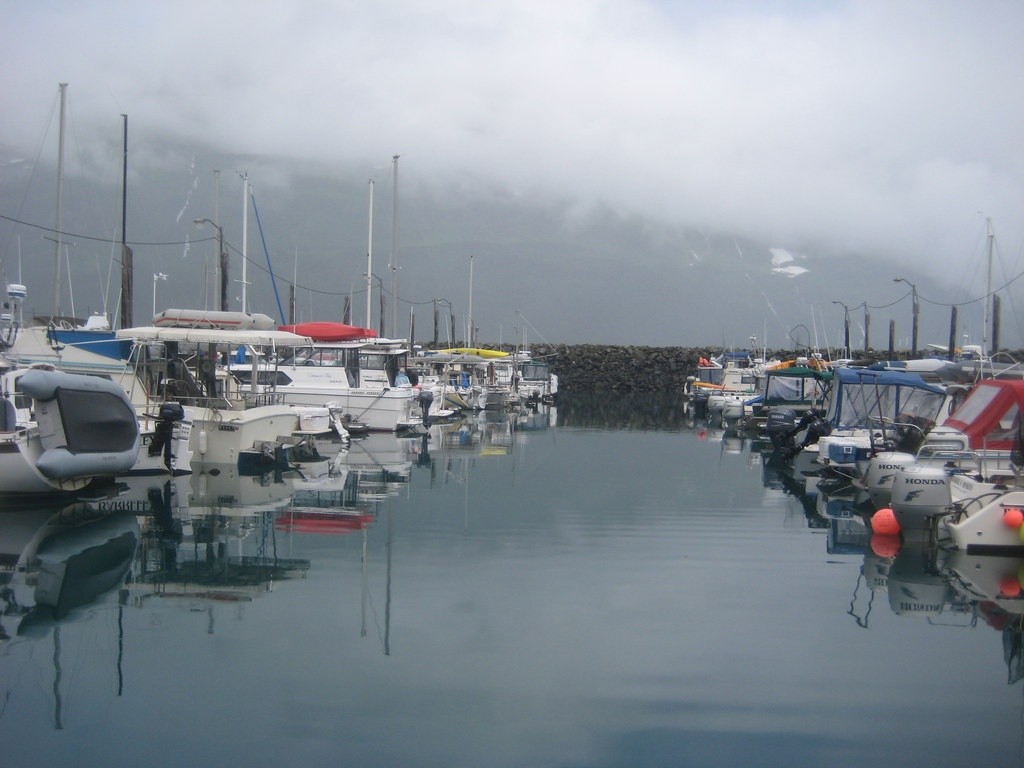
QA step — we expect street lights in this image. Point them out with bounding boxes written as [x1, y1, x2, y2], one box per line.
[831, 300, 853, 360]
[893, 277, 919, 359]
[361, 271, 386, 338]
[193, 216, 228, 312]
[437, 298, 456, 348]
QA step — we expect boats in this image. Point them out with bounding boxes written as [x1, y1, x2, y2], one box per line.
[681, 349, 1024, 561]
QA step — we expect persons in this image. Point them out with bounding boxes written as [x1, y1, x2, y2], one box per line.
[394, 366, 411, 388]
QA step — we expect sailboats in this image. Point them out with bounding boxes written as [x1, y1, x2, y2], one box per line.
[1, 78, 564, 502]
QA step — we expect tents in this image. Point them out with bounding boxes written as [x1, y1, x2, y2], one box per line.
[426, 347, 510, 359]
[277, 321, 378, 342]
